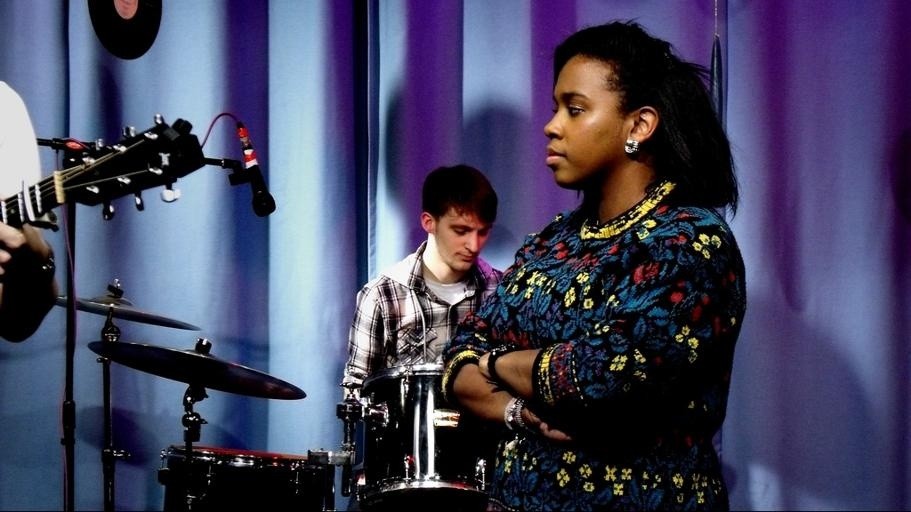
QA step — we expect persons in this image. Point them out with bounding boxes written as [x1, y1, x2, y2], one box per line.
[438, 15, 750, 510]
[0, 77, 58, 344]
[338, 162, 511, 489]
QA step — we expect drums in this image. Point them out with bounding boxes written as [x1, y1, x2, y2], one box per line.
[158, 444, 336, 512]
[347, 363, 497, 511]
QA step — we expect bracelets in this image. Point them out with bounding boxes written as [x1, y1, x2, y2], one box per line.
[504, 396, 527, 432]
[487, 341, 522, 394]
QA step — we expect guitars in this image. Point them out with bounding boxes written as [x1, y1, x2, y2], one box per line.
[0, 113, 205, 230]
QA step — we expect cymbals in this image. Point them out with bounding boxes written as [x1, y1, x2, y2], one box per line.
[54, 295, 201, 331]
[87, 342, 306, 400]
